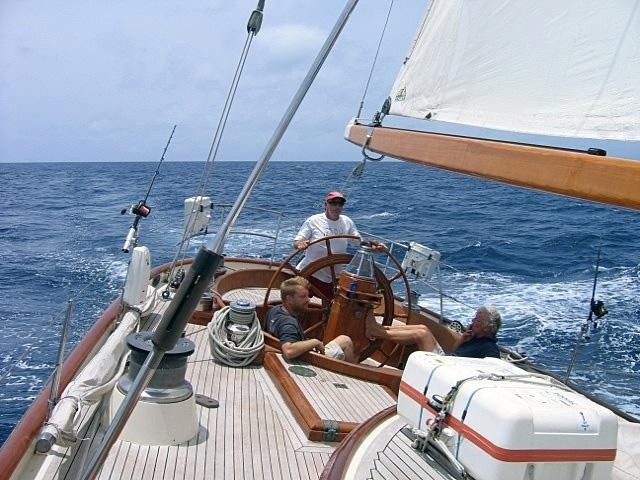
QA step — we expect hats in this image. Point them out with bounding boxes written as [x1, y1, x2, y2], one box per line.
[326, 191, 346, 203]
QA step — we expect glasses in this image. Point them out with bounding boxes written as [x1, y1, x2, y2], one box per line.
[329, 201, 344, 206]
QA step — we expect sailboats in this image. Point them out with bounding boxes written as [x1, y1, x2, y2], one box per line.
[1, 0, 640, 478]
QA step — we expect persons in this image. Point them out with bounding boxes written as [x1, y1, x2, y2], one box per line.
[263, 275, 355, 364]
[294, 192, 388, 285]
[366, 305, 502, 360]
